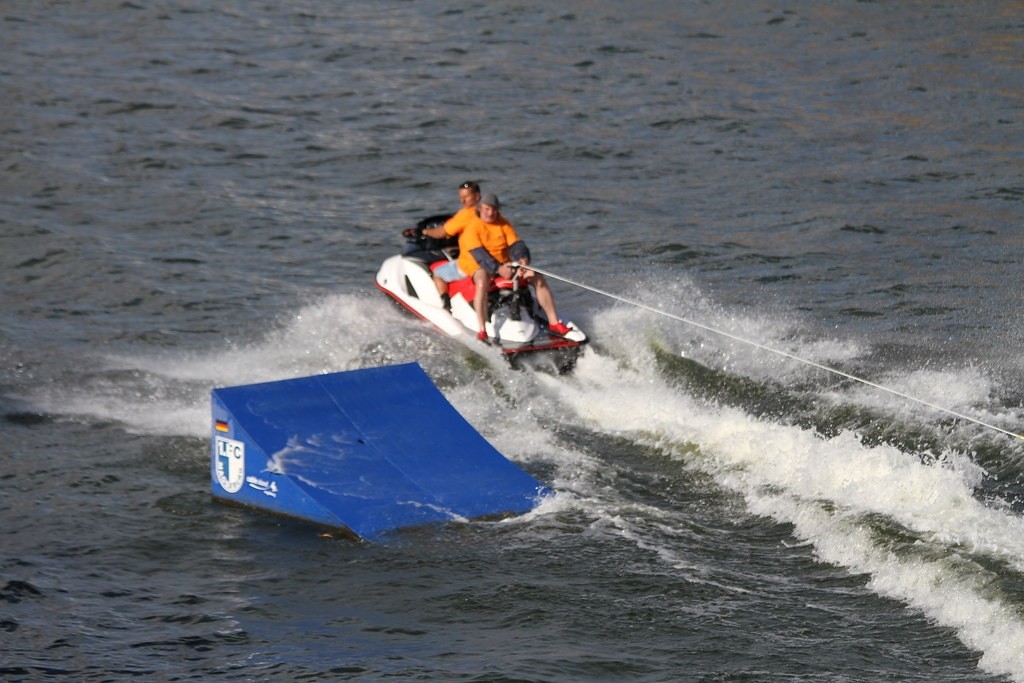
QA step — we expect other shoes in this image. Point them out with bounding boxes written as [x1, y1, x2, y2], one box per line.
[443, 297, 454, 314]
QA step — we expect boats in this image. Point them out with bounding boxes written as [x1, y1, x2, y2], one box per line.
[373, 213, 589, 356]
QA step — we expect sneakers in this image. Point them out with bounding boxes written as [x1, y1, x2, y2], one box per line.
[477, 330, 489, 341]
[548, 320, 572, 337]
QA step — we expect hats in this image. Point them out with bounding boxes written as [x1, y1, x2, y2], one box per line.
[482, 193, 502, 207]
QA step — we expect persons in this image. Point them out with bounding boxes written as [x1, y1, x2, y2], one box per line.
[422, 181, 482, 314]
[465, 193, 573, 341]
